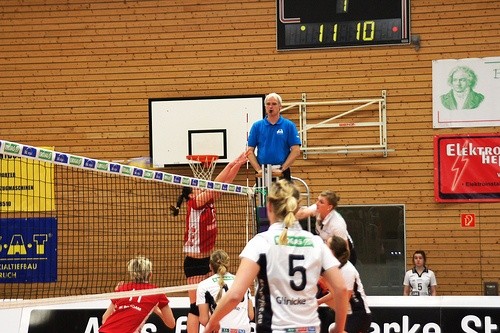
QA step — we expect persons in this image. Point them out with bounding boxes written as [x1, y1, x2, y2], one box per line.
[246, 92, 301, 208]
[98, 256, 175, 333]
[293, 188, 373, 333]
[337, 207, 387, 278]
[195, 249, 254, 333]
[403, 250, 437, 297]
[204, 179, 348, 333]
[169, 148, 251, 333]
